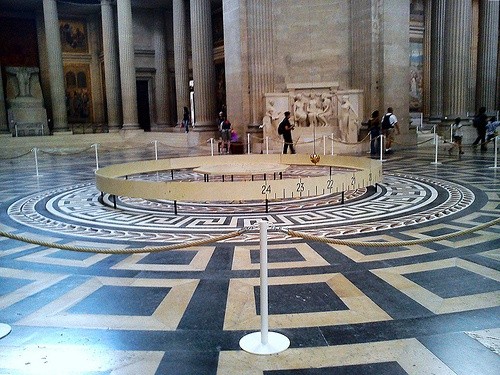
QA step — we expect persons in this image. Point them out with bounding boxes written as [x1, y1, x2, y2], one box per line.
[368, 107, 401, 157]
[266, 92, 358, 144]
[279, 112, 297, 154]
[182, 106, 190, 133]
[447, 107, 500, 156]
[216, 105, 233, 155]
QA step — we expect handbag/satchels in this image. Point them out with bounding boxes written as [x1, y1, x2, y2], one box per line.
[472, 120, 477, 128]
[494, 131, 499, 136]
[180, 123, 184, 128]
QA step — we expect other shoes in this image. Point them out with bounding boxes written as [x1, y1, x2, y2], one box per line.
[459, 151, 464, 154]
[386, 150, 393, 155]
[448, 149, 452, 156]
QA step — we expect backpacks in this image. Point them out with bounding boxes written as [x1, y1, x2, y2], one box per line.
[278, 122, 286, 135]
[222, 121, 231, 130]
[381, 113, 395, 130]
